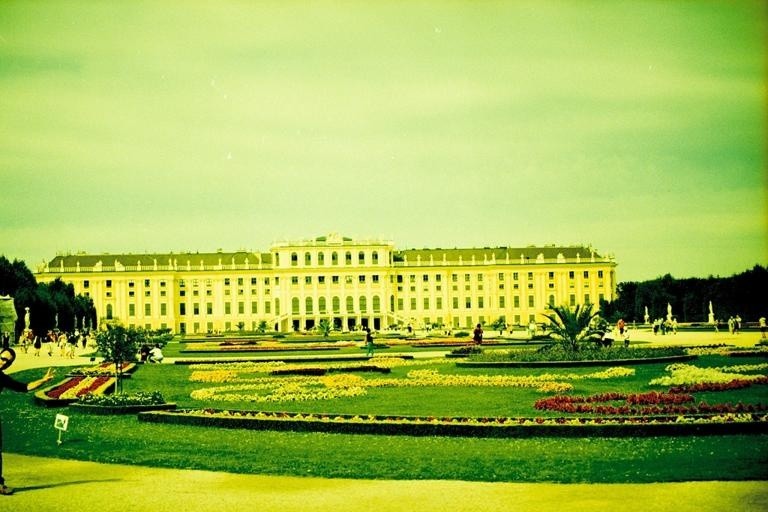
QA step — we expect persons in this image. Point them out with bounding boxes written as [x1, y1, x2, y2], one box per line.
[473, 323, 484, 347]
[757, 315, 767, 339]
[650, 317, 679, 335]
[600, 326, 614, 349]
[0, 348, 57, 496]
[20, 328, 87, 359]
[622, 329, 630, 347]
[2, 331, 11, 349]
[713, 319, 720, 332]
[617, 318, 624, 335]
[138, 344, 155, 363]
[733, 314, 741, 334]
[528, 320, 535, 337]
[363, 327, 373, 356]
[727, 315, 735, 333]
[148, 343, 163, 363]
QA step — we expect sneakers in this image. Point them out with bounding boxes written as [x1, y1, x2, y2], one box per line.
[0, 484, 15, 495]
